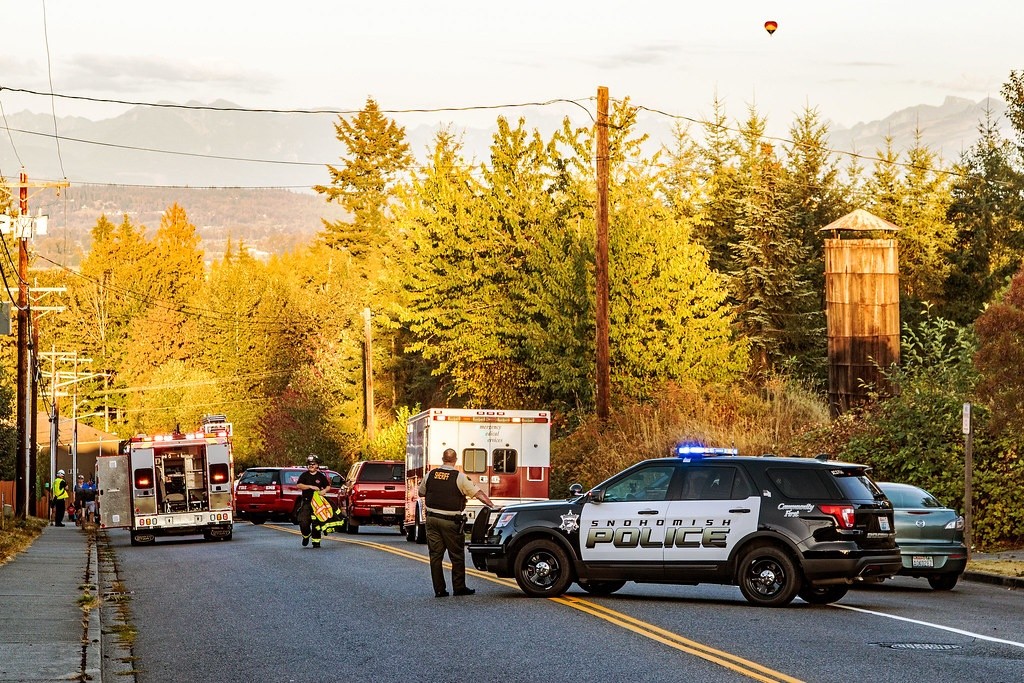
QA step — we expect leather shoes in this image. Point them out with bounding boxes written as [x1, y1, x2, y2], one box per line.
[435, 590, 449, 597]
[453, 587, 475, 596]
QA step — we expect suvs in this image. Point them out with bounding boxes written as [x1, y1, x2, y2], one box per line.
[235, 465, 346, 526]
[331, 460, 406, 535]
[467, 446, 903, 608]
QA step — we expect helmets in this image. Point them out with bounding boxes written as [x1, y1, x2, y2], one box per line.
[306, 455, 322, 465]
[78, 475, 84, 479]
[57, 470, 65, 475]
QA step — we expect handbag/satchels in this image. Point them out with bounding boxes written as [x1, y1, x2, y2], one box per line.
[51, 496, 57, 508]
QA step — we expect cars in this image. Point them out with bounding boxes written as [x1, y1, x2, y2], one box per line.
[874, 482, 967, 590]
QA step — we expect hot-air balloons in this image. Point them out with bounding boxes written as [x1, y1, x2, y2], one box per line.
[764, 20, 778, 35]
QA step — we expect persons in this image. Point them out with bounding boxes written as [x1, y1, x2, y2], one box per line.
[295, 454, 331, 548]
[418, 446, 495, 597]
[73, 475, 97, 523]
[50, 469, 70, 526]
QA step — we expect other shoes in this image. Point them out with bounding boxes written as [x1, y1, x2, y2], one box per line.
[312, 542, 320, 548]
[55, 522, 65, 526]
[302, 537, 309, 546]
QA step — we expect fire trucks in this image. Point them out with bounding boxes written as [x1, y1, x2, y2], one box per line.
[95, 414, 236, 547]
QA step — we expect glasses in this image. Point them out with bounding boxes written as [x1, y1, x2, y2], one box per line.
[308, 463, 316, 465]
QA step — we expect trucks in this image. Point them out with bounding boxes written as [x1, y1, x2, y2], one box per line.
[402, 407, 553, 545]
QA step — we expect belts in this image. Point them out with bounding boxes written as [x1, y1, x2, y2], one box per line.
[426, 512, 457, 521]
